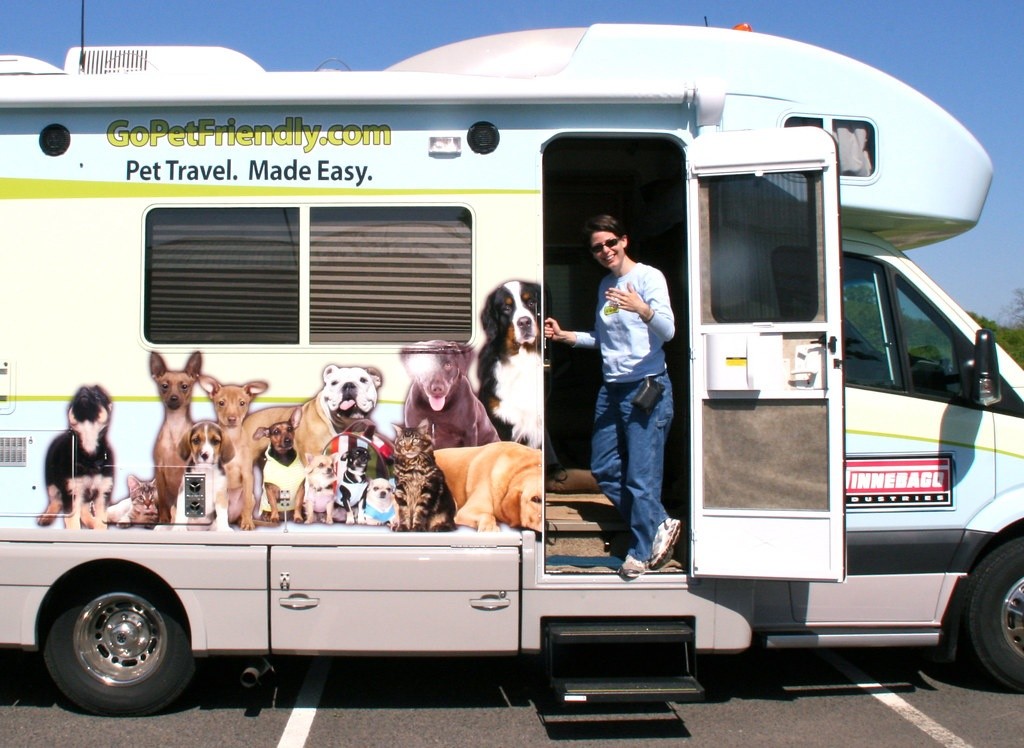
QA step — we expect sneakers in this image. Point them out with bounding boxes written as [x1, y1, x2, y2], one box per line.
[618, 552, 646, 579]
[649, 517, 681, 571]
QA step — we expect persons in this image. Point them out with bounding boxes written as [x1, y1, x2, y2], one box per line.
[544, 213, 681, 579]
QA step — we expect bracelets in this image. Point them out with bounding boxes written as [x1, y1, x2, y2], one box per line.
[643, 310, 655, 323]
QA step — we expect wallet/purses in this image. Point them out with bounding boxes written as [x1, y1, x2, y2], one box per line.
[631, 377, 666, 417]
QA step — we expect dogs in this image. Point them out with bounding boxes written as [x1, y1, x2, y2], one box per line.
[34, 279, 542, 532]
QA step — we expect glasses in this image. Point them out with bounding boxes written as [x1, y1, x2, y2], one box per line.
[590, 238, 621, 253]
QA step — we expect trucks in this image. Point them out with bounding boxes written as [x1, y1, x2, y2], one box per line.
[0, 16, 1024, 718]
